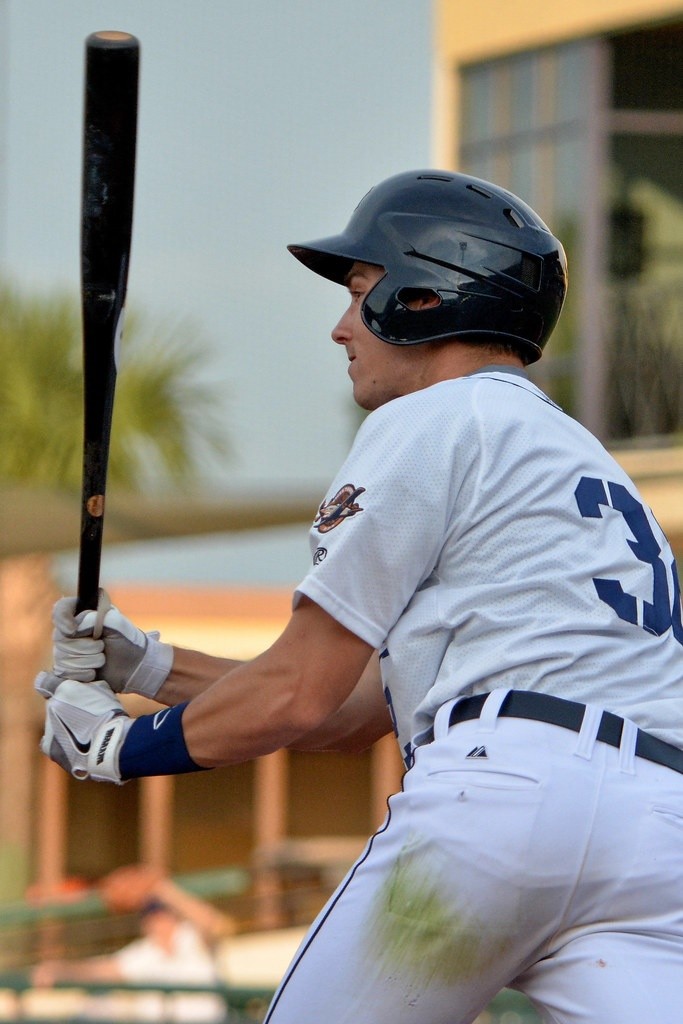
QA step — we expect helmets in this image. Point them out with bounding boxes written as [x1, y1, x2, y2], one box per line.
[286, 169, 567, 366]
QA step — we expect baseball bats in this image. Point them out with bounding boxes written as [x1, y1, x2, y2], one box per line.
[74, 32, 140, 681]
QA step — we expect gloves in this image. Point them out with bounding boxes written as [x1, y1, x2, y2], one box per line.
[33, 669, 136, 786]
[50, 586, 175, 701]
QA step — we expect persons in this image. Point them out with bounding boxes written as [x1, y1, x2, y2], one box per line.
[32, 168, 680, 1024]
[93, 868, 241, 1020]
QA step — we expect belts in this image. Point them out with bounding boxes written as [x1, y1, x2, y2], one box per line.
[424, 689, 683, 774]
